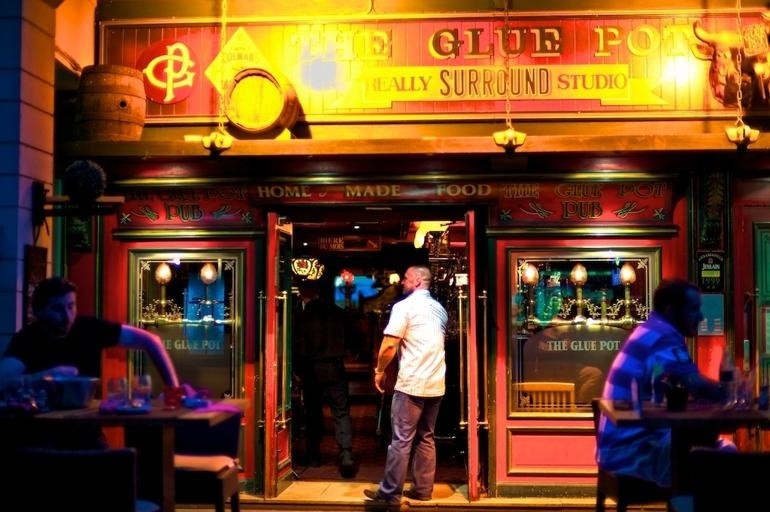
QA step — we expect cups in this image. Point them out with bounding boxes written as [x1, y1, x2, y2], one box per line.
[106, 372, 154, 404]
[665, 386, 689, 411]
[162, 384, 184, 411]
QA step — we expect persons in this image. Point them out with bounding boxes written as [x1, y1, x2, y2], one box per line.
[292, 280, 359, 478]
[0, 276, 198, 452]
[364, 266, 448, 508]
[593, 277, 756, 512]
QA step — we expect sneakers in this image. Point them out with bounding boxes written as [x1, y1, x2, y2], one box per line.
[364, 489, 401, 505]
[402, 490, 432, 500]
[340, 455, 355, 468]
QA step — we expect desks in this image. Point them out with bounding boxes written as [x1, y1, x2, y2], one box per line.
[597, 397, 769, 499]
[33, 398, 245, 511]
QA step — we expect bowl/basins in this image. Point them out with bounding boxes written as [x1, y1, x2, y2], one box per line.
[42, 374, 100, 408]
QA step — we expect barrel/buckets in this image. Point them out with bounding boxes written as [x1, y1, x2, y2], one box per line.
[74, 65, 147, 143]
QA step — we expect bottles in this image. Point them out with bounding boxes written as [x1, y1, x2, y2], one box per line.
[718, 347, 736, 405]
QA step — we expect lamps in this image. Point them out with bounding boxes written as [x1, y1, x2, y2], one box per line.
[619, 261, 637, 315]
[200, 0, 235, 154]
[200, 262, 218, 301]
[522, 262, 541, 319]
[154, 262, 171, 315]
[724, 0, 761, 147]
[570, 261, 589, 315]
[490, 1, 530, 153]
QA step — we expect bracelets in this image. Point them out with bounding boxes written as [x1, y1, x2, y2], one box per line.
[375, 368, 384, 374]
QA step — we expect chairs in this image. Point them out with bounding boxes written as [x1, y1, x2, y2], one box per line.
[0, 442, 157, 511]
[591, 397, 672, 512]
[686, 442, 769, 511]
[513, 382, 576, 408]
[174, 395, 251, 511]
